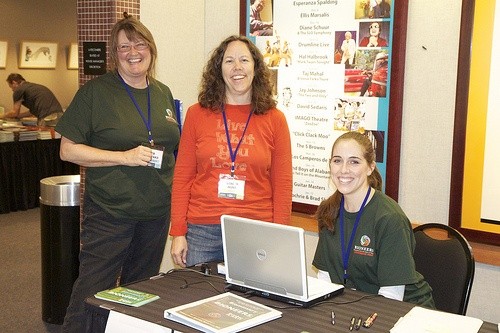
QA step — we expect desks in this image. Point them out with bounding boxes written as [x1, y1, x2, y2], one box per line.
[85, 259, 499, 333]
[0, 138, 80, 214]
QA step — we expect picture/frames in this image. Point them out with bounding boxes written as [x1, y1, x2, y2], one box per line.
[448, 0, 500, 248]
[19, 39, 59, 69]
[0, 40, 9, 69]
[67, 41, 78, 70]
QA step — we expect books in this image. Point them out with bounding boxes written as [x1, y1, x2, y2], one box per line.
[0, 118, 61, 141]
[93, 286, 160, 307]
[390, 305, 483, 333]
[164, 292, 283, 333]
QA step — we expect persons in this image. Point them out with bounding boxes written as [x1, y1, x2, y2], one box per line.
[264, 41, 292, 67]
[372, 0, 390, 18]
[54, 19, 180, 333]
[371, 54, 388, 97]
[312, 134, 435, 310]
[170, 34, 292, 268]
[341, 32, 356, 64]
[250, 0, 273, 36]
[360, 23, 386, 46]
[0, 73, 64, 126]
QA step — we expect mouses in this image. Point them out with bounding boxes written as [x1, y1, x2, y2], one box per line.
[202, 263, 213, 275]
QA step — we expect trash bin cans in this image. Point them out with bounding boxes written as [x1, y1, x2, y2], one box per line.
[37, 174, 81, 325]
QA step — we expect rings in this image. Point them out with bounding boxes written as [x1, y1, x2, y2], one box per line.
[146, 163, 148, 167]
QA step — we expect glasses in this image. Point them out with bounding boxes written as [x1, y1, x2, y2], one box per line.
[370, 25, 379, 28]
[116, 41, 148, 52]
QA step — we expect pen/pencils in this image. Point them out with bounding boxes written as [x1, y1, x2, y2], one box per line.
[366, 312, 377, 328]
[331, 310, 335, 325]
[356, 317, 362, 330]
[348, 317, 355, 331]
[363, 314, 373, 326]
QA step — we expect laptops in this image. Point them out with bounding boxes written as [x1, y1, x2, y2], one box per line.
[221, 213, 346, 309]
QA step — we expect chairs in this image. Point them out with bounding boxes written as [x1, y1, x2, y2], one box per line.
[411, 223, 475, 316]
